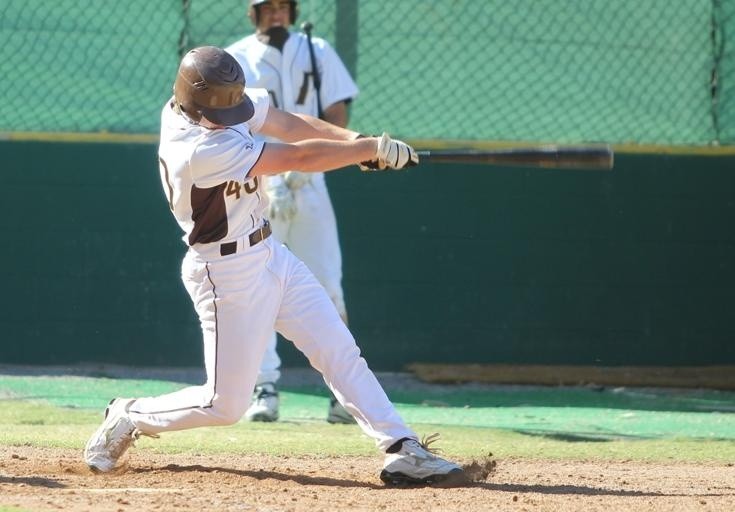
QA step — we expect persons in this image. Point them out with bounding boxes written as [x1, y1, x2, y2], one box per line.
[223, 1, 362, 423]
[82, 46, 468, 488]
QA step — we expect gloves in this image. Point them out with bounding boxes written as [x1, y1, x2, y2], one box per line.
[376, 134, 421, 171]
[352, 134, 379, 173]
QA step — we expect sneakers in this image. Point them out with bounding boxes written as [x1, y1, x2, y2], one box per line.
[82, 395, 164, 478]
[326, 396, 359, 424]
[379, 432, 466, 488]
[244, 382, 281, 423]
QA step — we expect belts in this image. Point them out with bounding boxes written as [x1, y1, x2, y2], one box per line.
[219, 219, 274, 257]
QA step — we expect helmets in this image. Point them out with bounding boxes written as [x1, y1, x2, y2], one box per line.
[172, 45, 257, 128]
[247, 0, 299, 27]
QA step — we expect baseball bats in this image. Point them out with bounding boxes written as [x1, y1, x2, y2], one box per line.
[302, 22, 325, 120]
[361, 138, 614, 171]
